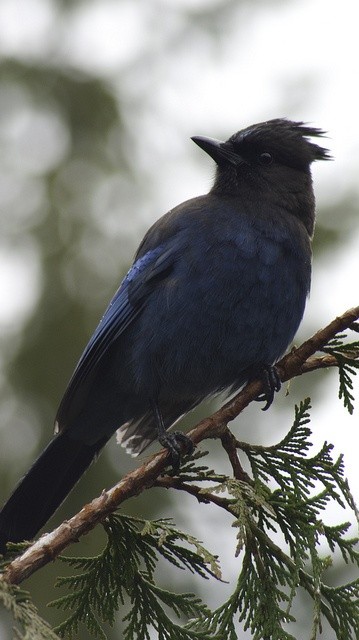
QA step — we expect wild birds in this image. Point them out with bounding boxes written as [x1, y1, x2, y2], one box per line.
[0, 117, 335, 563]
